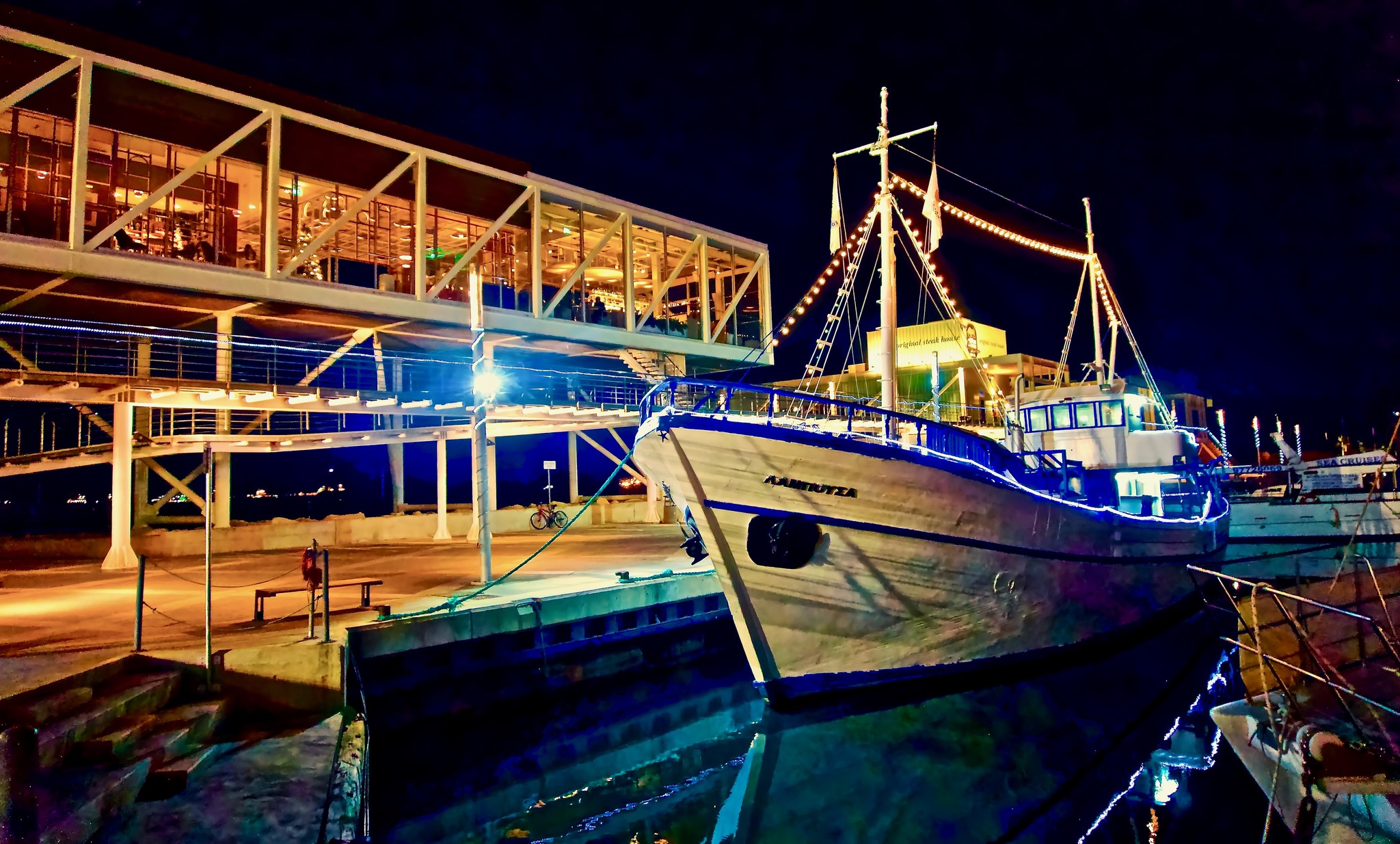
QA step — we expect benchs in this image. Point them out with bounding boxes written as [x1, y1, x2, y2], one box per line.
[252, 578, 382, 620]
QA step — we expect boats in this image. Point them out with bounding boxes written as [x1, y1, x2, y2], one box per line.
[635, 85, 1400, 844]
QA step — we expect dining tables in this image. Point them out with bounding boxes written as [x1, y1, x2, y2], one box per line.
[572, 305, 599, 323]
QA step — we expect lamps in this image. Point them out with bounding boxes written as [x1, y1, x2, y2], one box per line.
[1099, 384, 1111, 391]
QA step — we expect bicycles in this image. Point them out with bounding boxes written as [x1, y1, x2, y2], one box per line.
[530, 502, 568, 530]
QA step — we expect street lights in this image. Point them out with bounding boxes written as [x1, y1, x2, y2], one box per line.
[469, 329, 501, 587]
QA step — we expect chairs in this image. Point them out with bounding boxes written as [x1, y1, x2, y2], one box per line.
[600, 310, 612, 325]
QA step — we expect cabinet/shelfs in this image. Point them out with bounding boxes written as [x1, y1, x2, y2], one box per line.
[115, 202, 216, 262]
